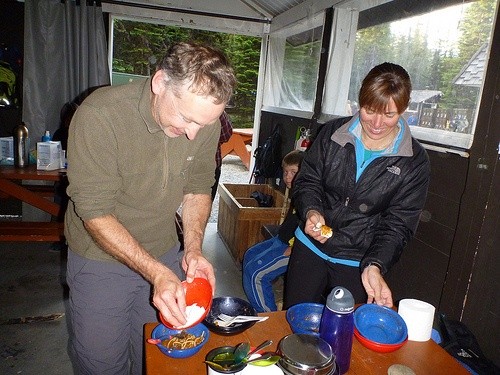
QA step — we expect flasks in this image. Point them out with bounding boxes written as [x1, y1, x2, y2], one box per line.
[13, 121, 28, 168]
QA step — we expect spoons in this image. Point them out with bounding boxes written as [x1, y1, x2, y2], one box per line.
[147, 334, 188, 344]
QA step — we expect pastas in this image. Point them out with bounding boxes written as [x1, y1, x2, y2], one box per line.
[167, 331, 204, 349]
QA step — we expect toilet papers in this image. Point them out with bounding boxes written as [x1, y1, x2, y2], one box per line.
[397, 298, 436, 343]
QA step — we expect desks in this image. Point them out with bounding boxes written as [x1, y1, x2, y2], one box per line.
[218, 129, 253, 169]
[0, 164, 64, 241]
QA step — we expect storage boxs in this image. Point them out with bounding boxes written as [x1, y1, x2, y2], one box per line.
[37, 141, 62, 170]
[218, 183, 292, 271]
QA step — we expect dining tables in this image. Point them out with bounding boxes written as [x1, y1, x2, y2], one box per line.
[144, 303, 472, 375]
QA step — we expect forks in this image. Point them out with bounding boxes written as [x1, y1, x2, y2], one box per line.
[214, 313, 269, 327]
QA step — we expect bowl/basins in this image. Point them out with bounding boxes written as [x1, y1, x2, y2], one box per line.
[204, 346, 247, 374]
[159, 277, 212, 330]
[152, 323, 209, 358]
[353, 303, 408, 353]
[201, 295, 258, 336]
[286, 303, 324, 336]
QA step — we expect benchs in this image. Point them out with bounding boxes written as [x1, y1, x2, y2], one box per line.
[0, 220, 64, 243]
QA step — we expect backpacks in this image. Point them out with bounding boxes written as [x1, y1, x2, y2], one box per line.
[253, 123, 283, 179]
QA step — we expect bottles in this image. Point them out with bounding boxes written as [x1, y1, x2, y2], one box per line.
[276, 334, 337, 375]
[319, 286, 355, 375]
[42, 130, 52, 142]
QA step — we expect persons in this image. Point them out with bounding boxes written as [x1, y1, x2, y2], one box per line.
[240, 149, 304, 311]
[64, 40, 239, 374]
[175, 109, 233, 252]
[346, 100, 358, 116]
[278, 61, 431, 308]
[449, 115, 469, 133]
[405, 112, 418, 126]
